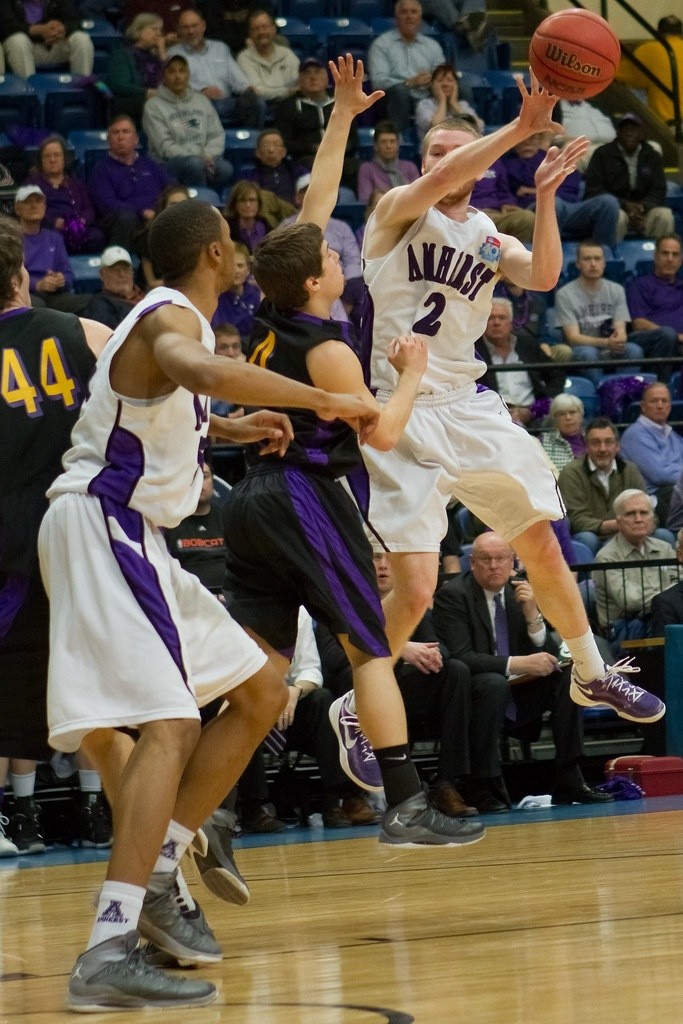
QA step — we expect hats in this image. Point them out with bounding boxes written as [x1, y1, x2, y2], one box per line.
[13, 184, 44, 205]
[619, 114, 643, 130]
[295, 174, 313, 194]
[298, 57, 324, 72]
[98, 245, 132, 271]
[164, 54, 185, 68]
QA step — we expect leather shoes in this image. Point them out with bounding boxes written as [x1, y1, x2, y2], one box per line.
[344, 796, 384, 822]
[475, 795, 509, 815]
[431, 781, 479, 818]
[551, 783, 614, 804]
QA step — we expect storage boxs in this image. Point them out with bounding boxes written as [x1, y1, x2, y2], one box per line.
[604, 755, 682, 796]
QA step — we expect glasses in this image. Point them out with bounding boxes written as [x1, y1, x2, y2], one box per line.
[474, 551, 512, 565]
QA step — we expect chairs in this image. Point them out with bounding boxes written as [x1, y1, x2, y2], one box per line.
[0, 0, 683, 812]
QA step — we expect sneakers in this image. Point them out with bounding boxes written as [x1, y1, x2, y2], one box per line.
[0, 814, 21, 856]
[321, 805, 351, 828]
[61, 790, 116, 848]
[127, 900, 219, 970]
[377, 791, 485, 850]
[10, 803, 49, 854]
[569, 655, 665, 722]
[65, 932, 218, 1011]
[329, 688, 386, 792]
[246, 812, 286, 832]
[189, 809, 251, 906]
[93, 871, 222, 963]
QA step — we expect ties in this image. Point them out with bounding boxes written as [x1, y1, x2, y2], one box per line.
[491, 594, 510, 661]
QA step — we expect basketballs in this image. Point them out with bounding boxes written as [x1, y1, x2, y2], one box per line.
[527, 8, 622, 102]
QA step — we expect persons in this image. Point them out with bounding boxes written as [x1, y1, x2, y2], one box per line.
[505, 129, 620, 242]
[329, 66, 666, 795]
[454, 113, 536, 242]
[275, 57, 365, 198]
[14, 174, 683, 855]
[237, 11, 301, 101]
[142, 54, 234, 189]
[0, 231, 215, 970]
[367, 0, 445, 128]
[415, 65, 485, 142]
[240, 129, 310, 205]
[101, 13, 167, 130]
[24, 133, 121, 258]
[37, 199, 380, 1013]
[166, 11, 268, 130]
[585, 114, 675, 241]
[358, 122, 421, 202]
[183, 53, 487, 905]
[87, 115, 179, 248]
[0, 0, 95, 81]
